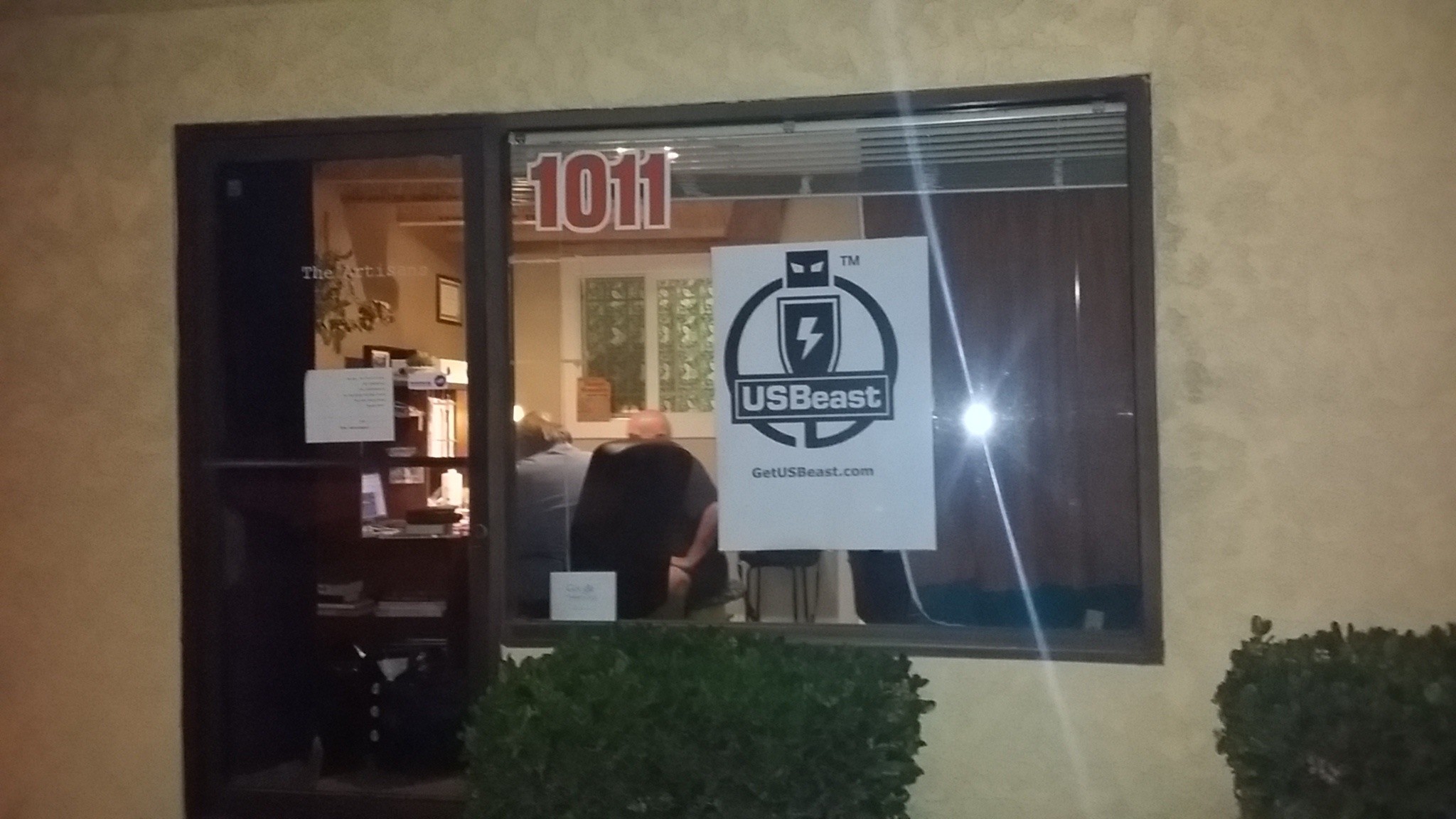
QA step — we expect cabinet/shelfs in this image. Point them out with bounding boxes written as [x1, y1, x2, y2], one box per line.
[361, 343, 474, 524]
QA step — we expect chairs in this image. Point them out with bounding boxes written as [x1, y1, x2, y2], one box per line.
[733, 546, 839, 628]
[685, 575, 751, 626]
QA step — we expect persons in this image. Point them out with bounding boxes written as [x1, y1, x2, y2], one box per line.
[627, 407, 727, 623]
[507, 410, 593, 621]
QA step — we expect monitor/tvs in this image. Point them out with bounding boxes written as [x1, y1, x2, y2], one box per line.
[462, 77, 1160, 650]
